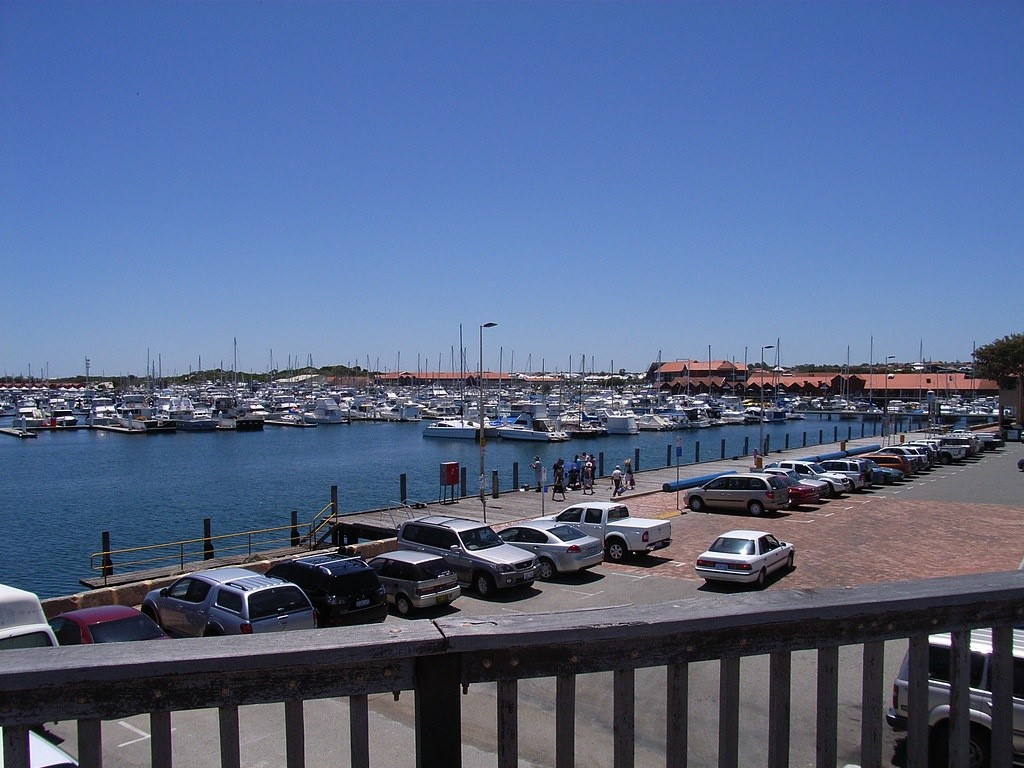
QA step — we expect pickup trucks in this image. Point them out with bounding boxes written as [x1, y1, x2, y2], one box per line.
[529, 501, 672, 563]
[142, 567, 320, 636]
[915, 439, 969, 465]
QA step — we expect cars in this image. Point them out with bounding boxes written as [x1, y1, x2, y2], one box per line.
[495, 523, 605, 580]
[839, 430, 1004, 487]
[743, 467, 828, 506]
[366, 552, 463, 616]
[45, 605, 171, 644]
[682, 471, 794, 517]
[694, 531, 795, 586]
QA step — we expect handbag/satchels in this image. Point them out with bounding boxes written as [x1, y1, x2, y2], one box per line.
[630, 479, 635, 486]
[617, 485, 626, 496]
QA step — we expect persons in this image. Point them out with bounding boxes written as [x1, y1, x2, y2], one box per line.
[528, 451, 597, 501]
[611, 459, 635, 497]
[753, 448, 759, 464]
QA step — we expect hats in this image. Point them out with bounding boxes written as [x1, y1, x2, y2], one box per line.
[585, 462, 592, 467]
[589, 453, 595, 458]
[534, 455, 541, 460]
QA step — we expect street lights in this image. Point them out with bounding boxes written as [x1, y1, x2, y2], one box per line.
[886, 355, 896, 441]
[479, 321, 499, 496]
[759, 345, 774, 459]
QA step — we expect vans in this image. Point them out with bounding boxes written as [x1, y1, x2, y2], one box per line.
[0, 581, 61, 650]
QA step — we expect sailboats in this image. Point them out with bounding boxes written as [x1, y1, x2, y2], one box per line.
[1, 335, 998, 441]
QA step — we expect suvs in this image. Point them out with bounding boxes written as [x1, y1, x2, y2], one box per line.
[402, 514, 544, 596]
[884, 624, 1023, 767]
[268, 552, 389, 623]
[820, 461, 874, 492]
[777, 461, 850, 498]
